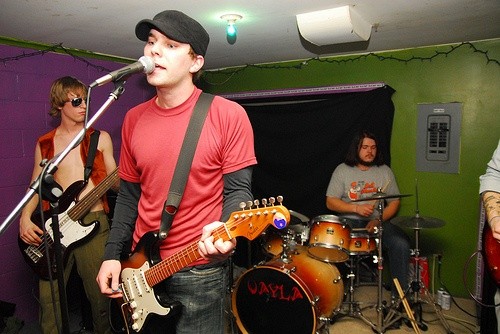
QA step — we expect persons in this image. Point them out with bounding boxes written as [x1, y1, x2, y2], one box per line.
[326, 129, 409, 306]
[480, 139, 500, 334]
[19, 76, 119, 334]
[96, 11, 258, 334]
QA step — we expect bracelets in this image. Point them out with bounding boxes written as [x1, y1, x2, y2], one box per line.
[356, 204, 358, 213]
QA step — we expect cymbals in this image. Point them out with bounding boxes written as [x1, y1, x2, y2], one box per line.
[348, 191, 414, 204]
[389, 214, 448, 229]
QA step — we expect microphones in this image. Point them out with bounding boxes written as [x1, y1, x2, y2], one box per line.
[90, 56, 155, 88]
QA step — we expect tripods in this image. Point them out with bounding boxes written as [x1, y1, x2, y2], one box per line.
[331, 198, 453, 334]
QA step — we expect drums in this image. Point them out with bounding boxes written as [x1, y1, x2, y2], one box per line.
[230, 249, 344, 334]
[349, 232, 380, 257]
[306, 213, 351, 264]
[258, 219, 295, 260]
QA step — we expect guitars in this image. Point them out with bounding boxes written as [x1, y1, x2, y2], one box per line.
[106, 194, 292, 334]
[17, 165, 122, 282]
[480, 216, 500, 284]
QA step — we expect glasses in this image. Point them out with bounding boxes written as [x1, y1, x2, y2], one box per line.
[63, 96, 91, 107]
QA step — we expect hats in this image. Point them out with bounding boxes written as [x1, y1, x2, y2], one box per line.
[134, 10, 210, 57]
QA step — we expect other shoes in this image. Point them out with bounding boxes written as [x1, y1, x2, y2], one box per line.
[399, 307, 428, 331]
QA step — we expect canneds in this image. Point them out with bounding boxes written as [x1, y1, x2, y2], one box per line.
[438, 288, 451, 310]
